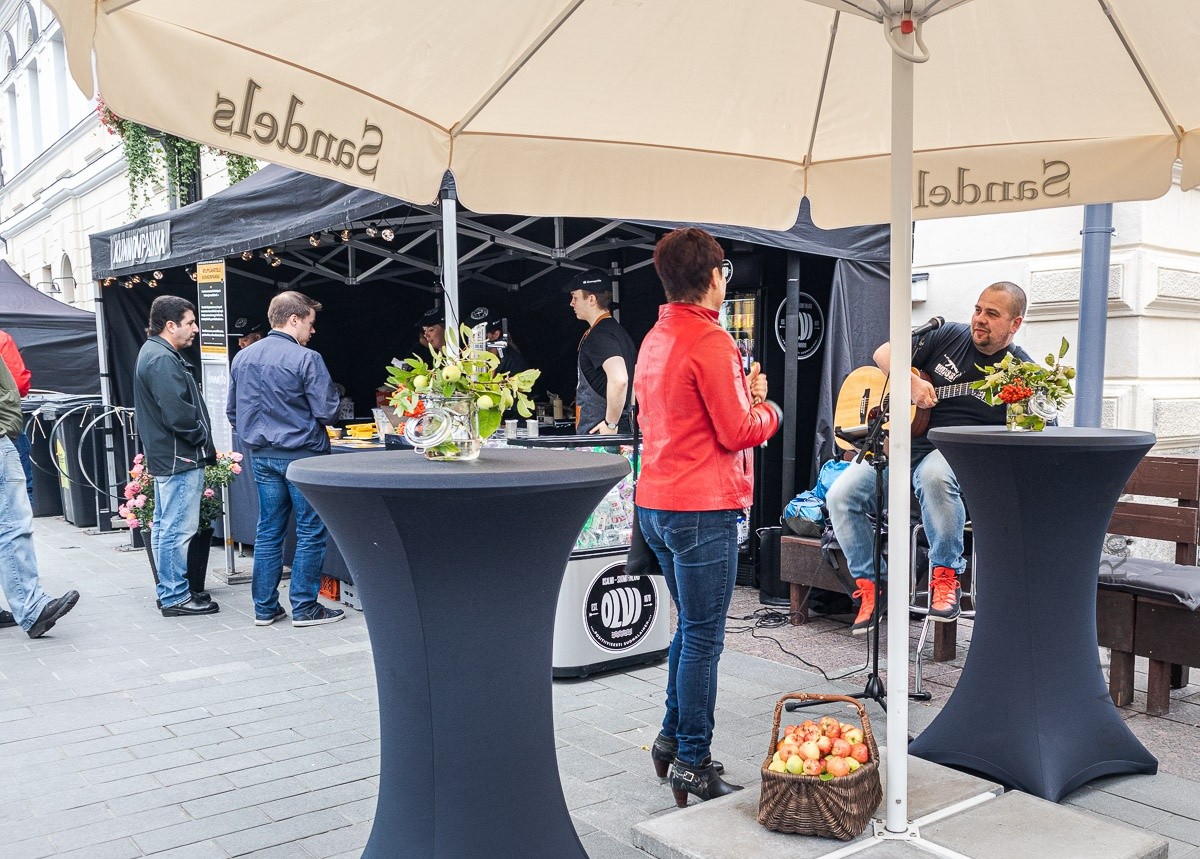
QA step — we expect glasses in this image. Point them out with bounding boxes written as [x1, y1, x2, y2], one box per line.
[719, 263, 729, 277]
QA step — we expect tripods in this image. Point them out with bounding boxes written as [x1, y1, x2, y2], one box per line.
[787, 341, 931, 743]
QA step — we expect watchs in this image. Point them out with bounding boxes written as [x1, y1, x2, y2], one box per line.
[605, 418, 618, 429]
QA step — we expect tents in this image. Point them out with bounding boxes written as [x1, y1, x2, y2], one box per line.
[0, 258, 105, 518]
[88, 162, 915, 587]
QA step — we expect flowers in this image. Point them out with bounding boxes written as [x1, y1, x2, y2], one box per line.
[973, 335, 1082, 423]
[114, 449, 244, 530]
[386, 326, 541, 443]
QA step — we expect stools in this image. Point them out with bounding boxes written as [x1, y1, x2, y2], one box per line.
[869, 508, 976, 705]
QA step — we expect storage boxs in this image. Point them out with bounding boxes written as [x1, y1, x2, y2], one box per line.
[374, 386, 395, 407]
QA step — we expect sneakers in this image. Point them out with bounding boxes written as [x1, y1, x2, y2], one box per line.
[851, 578, 888, 635]
[926, 567, 962, 621]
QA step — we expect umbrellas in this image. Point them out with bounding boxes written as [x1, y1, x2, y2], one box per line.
[43, 0, 1200, 841]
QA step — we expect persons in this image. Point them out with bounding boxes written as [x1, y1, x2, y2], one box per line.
[227, 290, 347, 624]
[0, 330, 35, 515]
[566, 269, 628, 438]
[231, 308, 536, 429]
[134, 299, 217, 614]
[0, 354, 79, 640]
[823, 282, 1053, 631]
[636, 229, 783, 806]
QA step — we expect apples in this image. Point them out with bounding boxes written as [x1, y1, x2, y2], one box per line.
[1011, 367, 1074, 413]
[768, 715, 869, 779]
[413, 365, 514, 410]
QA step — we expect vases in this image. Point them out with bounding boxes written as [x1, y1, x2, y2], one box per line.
[140, 527, 215, 595]
[398, 395, 485, 466]
[1002, 400, 1058, 432]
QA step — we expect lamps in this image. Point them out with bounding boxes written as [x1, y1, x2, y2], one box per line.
[365, 222, 379, 238]
[339, 228, 354, 243]
[142, 275, 159, 290]
[381, 223, 401, 244]
[184, 266, 197, 282]
[261, 248, 282, 269]
[117, 275, 141, 288]
[309, 232, 322, 247]
[152, 269, 166, 280]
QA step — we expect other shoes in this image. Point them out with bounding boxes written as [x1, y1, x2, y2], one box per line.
[25, 590, 80, 639]
[292, 602, 345, 627]
[255, 602, 286, 626]
[0, 610, 18, 627]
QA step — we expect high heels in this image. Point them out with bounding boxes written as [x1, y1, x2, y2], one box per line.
[652, 732, 724, 783]
[669, 752, 745, 807]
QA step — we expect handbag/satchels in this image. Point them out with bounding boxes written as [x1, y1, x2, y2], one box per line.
[622, 480, 663, 575]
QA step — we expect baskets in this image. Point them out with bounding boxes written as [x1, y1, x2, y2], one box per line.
[756, 693, 883, 840]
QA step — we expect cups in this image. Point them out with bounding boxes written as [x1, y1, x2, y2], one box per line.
[553, 395, 563, 419]
[326, 426, 343, 439]
[526, 419, 539, 438]
[505, 420, 518, 438]
[371, 408, 394, 443]
[544, 417, 554, 424]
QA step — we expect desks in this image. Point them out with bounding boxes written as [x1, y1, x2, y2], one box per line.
[908, 425, 1162, 804]
[279, 445, 636, 859]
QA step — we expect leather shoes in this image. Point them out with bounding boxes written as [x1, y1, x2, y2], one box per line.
[156, 590, 211, 609]
[160, 596, 219, 617]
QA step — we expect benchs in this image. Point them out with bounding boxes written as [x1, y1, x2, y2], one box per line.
[778, 452, 1199, 719]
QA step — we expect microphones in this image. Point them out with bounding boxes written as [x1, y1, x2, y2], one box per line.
[912, 316, 945, 337]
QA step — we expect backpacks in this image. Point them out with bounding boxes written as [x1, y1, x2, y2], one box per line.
[781, 455, 853, 538]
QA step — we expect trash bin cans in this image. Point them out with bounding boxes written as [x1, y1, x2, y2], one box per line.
[17, 393, 87, 518]
[41, 400, 109, 528]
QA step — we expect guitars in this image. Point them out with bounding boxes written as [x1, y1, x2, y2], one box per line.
[833, 365, 980, 451]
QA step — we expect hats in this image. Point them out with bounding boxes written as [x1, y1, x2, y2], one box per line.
[465, 307, 503, 332]
[562, 268, 611, 293]
[419, 307, 445, 326]
